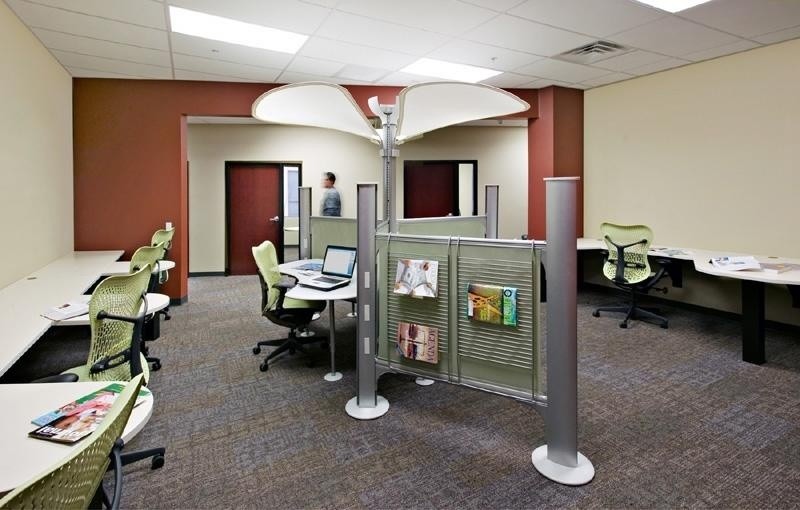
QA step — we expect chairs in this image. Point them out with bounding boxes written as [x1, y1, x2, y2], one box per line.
[251, 239, 327, 372]
[592, 222, 673, 329]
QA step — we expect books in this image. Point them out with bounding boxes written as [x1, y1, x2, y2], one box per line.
[396, 322, 439, 365]
[393, 258, 439, 299]
[27, 383, 152, 444]
[751, 262, 792, 274]
[467, 283, 519, 328]
[39, 301, 90, 322]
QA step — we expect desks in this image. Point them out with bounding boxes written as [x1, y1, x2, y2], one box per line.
[278, 258, 358, 382]
[577, 237, 800, 366]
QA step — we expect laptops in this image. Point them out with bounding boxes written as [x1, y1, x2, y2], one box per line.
[296, 245, 357, 291]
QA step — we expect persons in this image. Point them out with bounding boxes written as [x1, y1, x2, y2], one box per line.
[320, 171, 341, 216]
